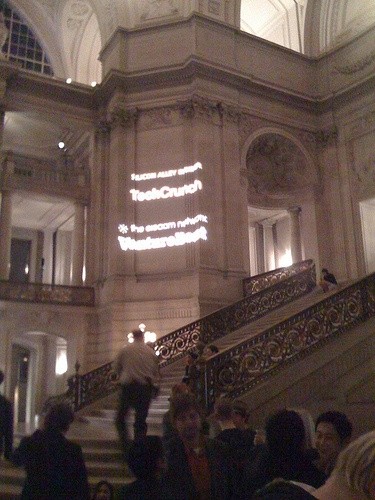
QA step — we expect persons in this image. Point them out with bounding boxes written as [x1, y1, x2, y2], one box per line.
[0, 346, 375, 500]
[114, 329, 160, 454]
[318, 268, 337, 292]
